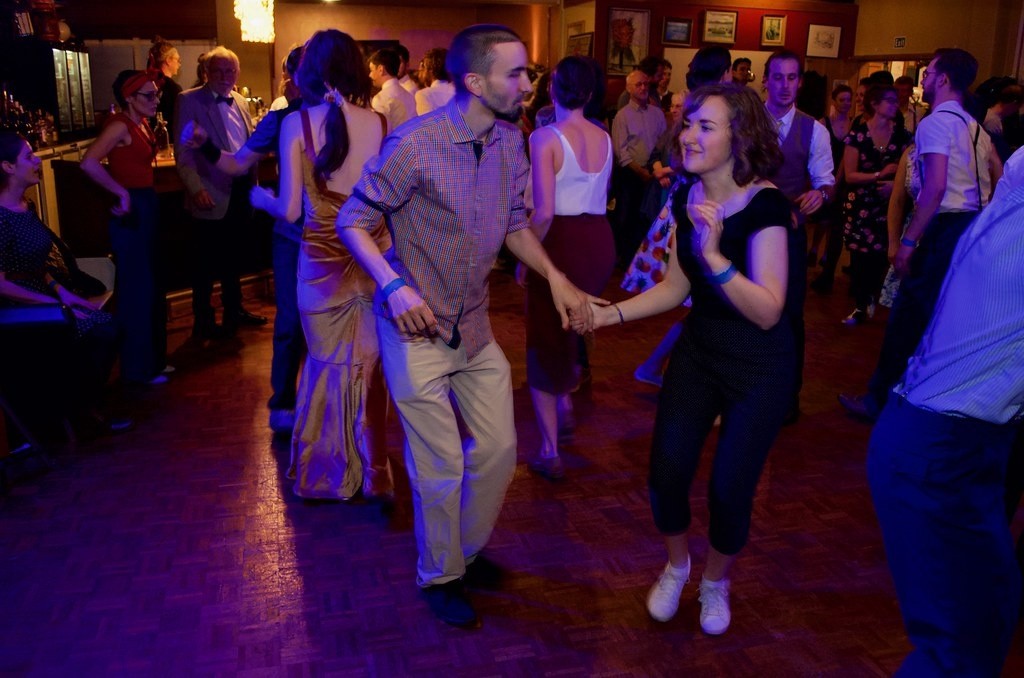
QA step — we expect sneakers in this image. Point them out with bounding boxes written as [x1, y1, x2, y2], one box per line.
[841, 310, 867, 325]
[698, 572, 731, 635]
[420, 577, 476, 627]
[865, 295, 875, 322]
[464, 555, 503, 590]
[646, 552, 691, 623]
[269, 408, 294, 432]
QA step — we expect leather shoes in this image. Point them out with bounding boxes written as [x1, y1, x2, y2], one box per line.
[76, 416, 136, 446]
[192, 324, 228, 339]
[837, 394, 876, 424]
[221, 312, 267, 327]
[781, 397, 799, 425]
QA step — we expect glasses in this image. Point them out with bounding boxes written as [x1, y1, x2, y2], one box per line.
[135, 90, 164, 101]
[168, 57, 182, 64]
[883, 96, 901, 105]
[923, 70, 942, 77]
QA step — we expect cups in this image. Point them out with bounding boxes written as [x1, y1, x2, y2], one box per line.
[745, 70, 756, 82]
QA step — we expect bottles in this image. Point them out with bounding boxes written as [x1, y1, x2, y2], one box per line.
[14, 0, 81, 47]
[234, 84, 265, 131]
[0, 86, 59, 152]
[154, 111, 171, 161]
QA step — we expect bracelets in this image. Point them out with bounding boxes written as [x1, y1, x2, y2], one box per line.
[821, 190, 829, 202]
[900, 237, 916, 247]
[198, 137, 220, 165]
[613, 304, 623, 325]
[875, 171, 880, 180]
[382, 278, 408, 299]
[49, 281, 58, 287]
[712, 262, 737, 284]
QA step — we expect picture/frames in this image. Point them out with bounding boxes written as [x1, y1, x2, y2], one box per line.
[661, 15, 692, 46]
[805, 24, 842, 59]
[605, 8, 653, 76]
[702, 10, 737, 45]
[760, 14, 786, 47]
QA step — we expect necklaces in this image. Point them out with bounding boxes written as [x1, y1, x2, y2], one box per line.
[880, 146, 882, 149]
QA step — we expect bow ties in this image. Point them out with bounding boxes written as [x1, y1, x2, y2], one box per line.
[215, 95, 234, 106]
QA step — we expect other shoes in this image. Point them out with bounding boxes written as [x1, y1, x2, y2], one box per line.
[807, 250, 817, 269]
[133, 374, 168, 386]
[161, 364, 175, 373]
[819, 258, 826, 266]
[366, 492, 396, 511]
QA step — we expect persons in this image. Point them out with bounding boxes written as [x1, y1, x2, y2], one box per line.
[0, 25, 1024, 678]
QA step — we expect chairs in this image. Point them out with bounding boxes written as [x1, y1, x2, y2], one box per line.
[0, 255, 117, 497]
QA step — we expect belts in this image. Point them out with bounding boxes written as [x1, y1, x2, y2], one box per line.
[887, 380, 1013, 442]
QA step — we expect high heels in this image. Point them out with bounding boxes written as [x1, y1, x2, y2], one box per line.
[526, 455, 563, 479]
[557, 425, 575, 443]
